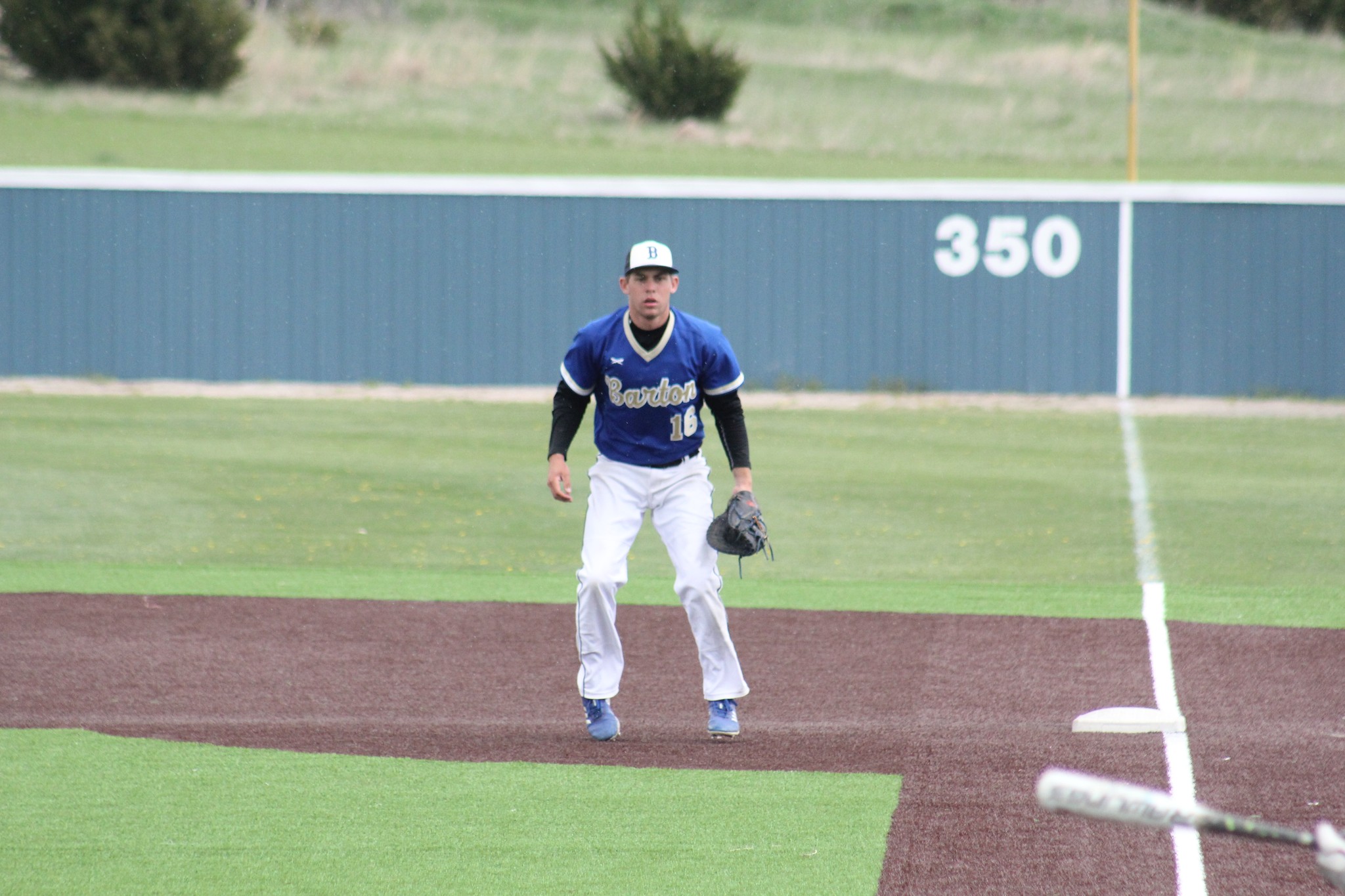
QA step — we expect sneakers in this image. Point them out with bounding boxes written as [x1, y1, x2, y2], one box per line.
[581, 697, 621, 741]
[708, 699, 740, 739]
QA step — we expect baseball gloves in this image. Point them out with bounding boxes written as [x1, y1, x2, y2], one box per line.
[706, 490, 767, 557]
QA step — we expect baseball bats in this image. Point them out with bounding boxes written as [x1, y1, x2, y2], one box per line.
[1034, 768, 1319, 850]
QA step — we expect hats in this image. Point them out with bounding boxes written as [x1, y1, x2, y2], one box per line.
[624, 241, 679, 275]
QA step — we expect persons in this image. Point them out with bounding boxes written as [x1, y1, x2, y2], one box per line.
[547, 239, 753, 741]
